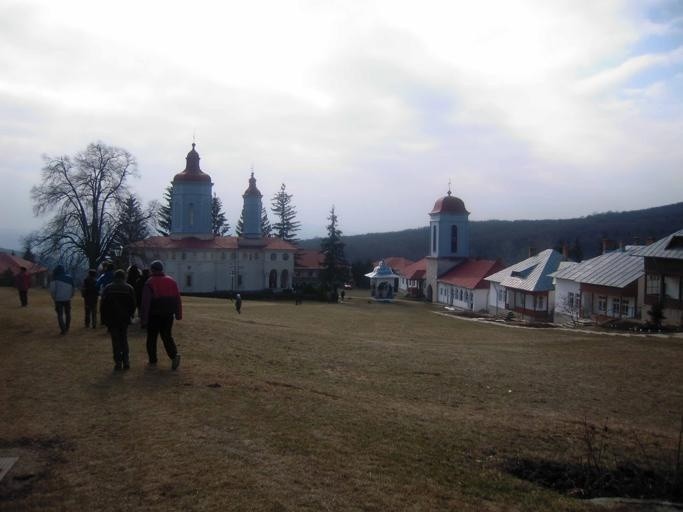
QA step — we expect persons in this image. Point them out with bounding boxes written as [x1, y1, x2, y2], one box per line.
[78, 260, 151, 331]
[138, 259, 183, 373]
[340, 289, 346, 302]
[50, 263, 75, 336]
[292, 280, 305, 305]
[234, 293, 242, 314]
[99, 269, 136, 372]
[14, 265, 33, 308]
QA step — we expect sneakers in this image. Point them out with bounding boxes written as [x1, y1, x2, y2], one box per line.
[113, 353, 158, 369]
[170, 354, 181, 370]
